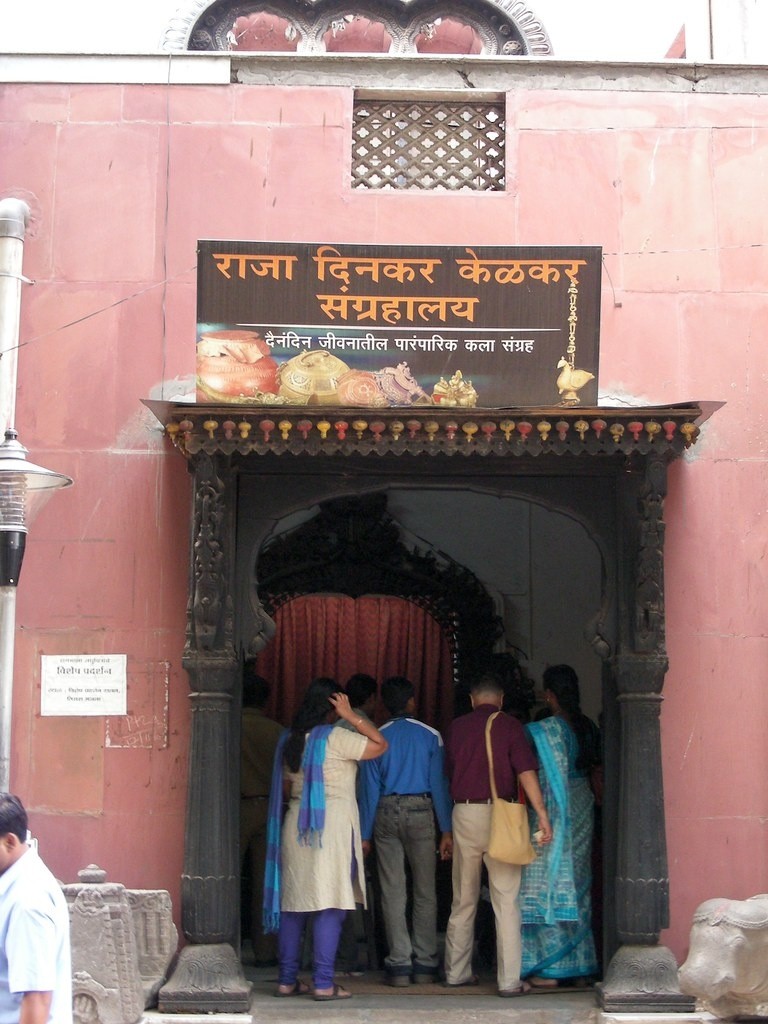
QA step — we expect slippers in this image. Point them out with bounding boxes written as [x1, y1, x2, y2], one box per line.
[527, 978, 557, 989]
[498, 985, 531, 996]
[275, 980, 314, 997]
[443, 975, 480, 987]
[314, 983, 352, 1000]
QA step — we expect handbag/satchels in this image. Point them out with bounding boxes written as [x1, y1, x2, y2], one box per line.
[488, 799, 537, 864]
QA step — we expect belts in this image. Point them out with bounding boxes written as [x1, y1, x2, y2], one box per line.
[455, 798, 517, 804]
[392, 792, 431, 799]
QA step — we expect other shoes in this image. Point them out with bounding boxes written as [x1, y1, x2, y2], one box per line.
[382, 966, 409, 986]
[255, 957, 278, 967]
[414, 966, 442, 983]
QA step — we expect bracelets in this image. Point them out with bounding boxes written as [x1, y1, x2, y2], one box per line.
[354, 718, 364, 728]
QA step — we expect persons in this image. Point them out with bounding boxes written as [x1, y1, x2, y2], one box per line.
[261, 675, 389, 1002]
[518, 664, 601, 992]
[334, 673, 379, 739]
[441, 675, 552, 996]
[358, 677, 453, 988]
[1, 792, 75, 1024]
